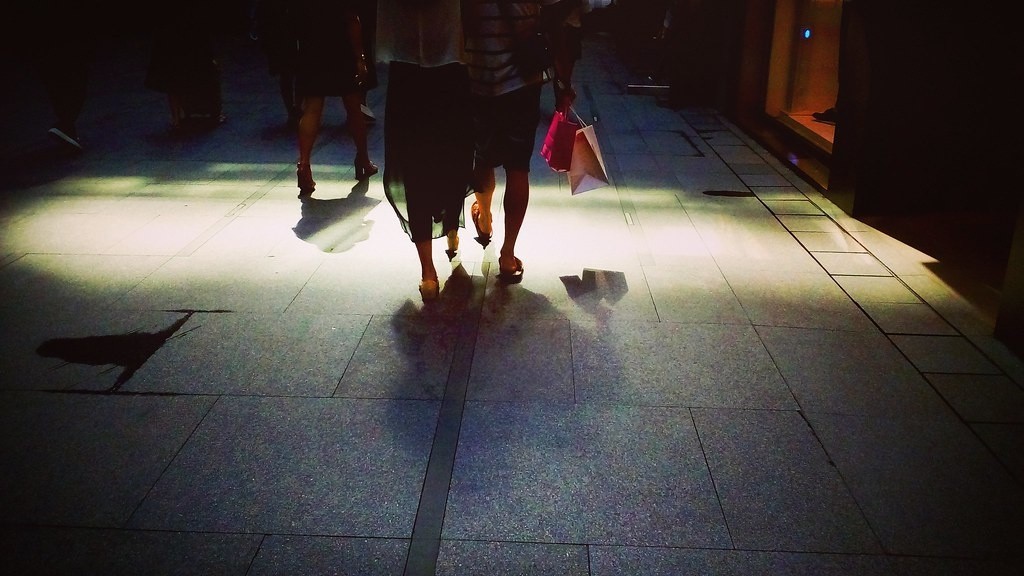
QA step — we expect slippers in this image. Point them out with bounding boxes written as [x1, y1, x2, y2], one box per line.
[471, 200, 494, 240]
[498, 256, 524, 284]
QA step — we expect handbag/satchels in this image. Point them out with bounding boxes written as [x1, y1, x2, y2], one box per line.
[539, 103, 610, 197]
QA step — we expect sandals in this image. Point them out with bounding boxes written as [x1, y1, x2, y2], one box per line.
[447, 235, 459, 250]
[419, 279, 441, 300]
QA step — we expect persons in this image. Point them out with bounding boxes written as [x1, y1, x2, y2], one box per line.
[276, 0, 378, 192]
[461, 0, 584, 283]
[374, 0, 483, 303]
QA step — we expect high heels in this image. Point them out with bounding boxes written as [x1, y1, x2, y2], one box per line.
[353, 161, 381, 181]
[295, 166, 315, 196]
